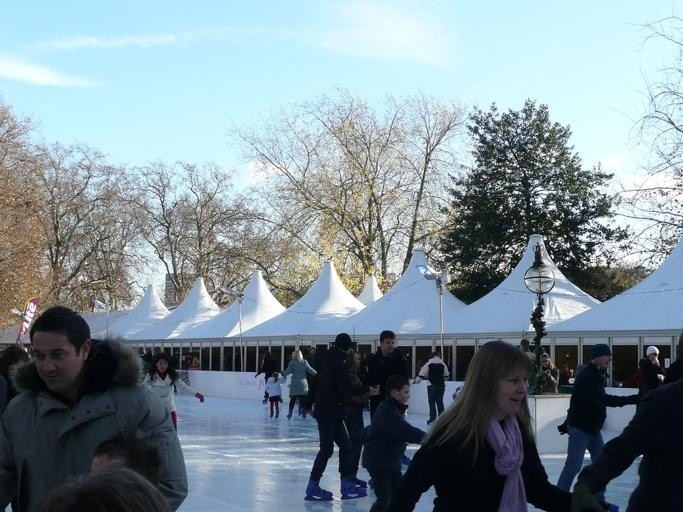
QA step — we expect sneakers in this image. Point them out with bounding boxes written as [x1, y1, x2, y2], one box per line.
[602, 499, 619, 511]
[269, 408, 313, 419]
[401, 454, 412, 465]
[427, 419, 435, 425]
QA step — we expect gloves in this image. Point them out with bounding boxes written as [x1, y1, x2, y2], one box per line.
[545, 370, 551, 378]
[558, 421, 568, 435]
[194, 392, 204, 401]
[625, 394, 638, 405]
[280, 371, 285, 375]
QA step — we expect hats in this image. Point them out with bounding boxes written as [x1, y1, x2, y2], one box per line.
[336, 333, 351, 350]
[590, 343, 611, 359]
[292, 349, 303, 360]
[542, 353, 551, 361]
[646, 347, 659, 356]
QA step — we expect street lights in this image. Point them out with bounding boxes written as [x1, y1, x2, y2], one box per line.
[218, 285, 245, 371]
[90, 297, 110, 338]
[417, 263, 445, 370]
[523, 242, 555, 374]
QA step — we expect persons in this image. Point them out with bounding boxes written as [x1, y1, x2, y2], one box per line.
[89, 435, 164, 485]
[32, 469, 170, 512]
[254, 330, 683, 512]
[0, 307, 188, 512]
[1, 346, 204, 435]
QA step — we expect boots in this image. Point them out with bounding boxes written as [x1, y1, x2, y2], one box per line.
[307, 478, 333, 499]
[340, 473, 367, 494]
